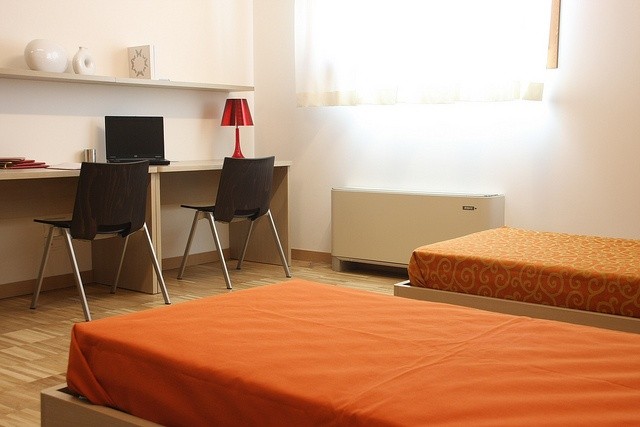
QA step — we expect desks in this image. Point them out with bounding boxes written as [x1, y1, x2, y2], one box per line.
[0, 162, 157, 295]
[157, 158, 294, 294]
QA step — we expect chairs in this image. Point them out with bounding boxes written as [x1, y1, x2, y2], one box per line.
[30, 161, 171, 321]
[176, 156, 291, 288]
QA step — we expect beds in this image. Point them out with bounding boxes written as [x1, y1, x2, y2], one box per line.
[39, 276, 639, 426]
[393, 224, 639, 334]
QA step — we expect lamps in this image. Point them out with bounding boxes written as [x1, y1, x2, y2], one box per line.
[219, 97, 254, 157]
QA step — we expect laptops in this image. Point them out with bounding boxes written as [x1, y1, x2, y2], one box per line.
[105, 115, 170, 165]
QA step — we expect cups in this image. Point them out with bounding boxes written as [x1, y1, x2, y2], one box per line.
[84, 148, 96, 162]
